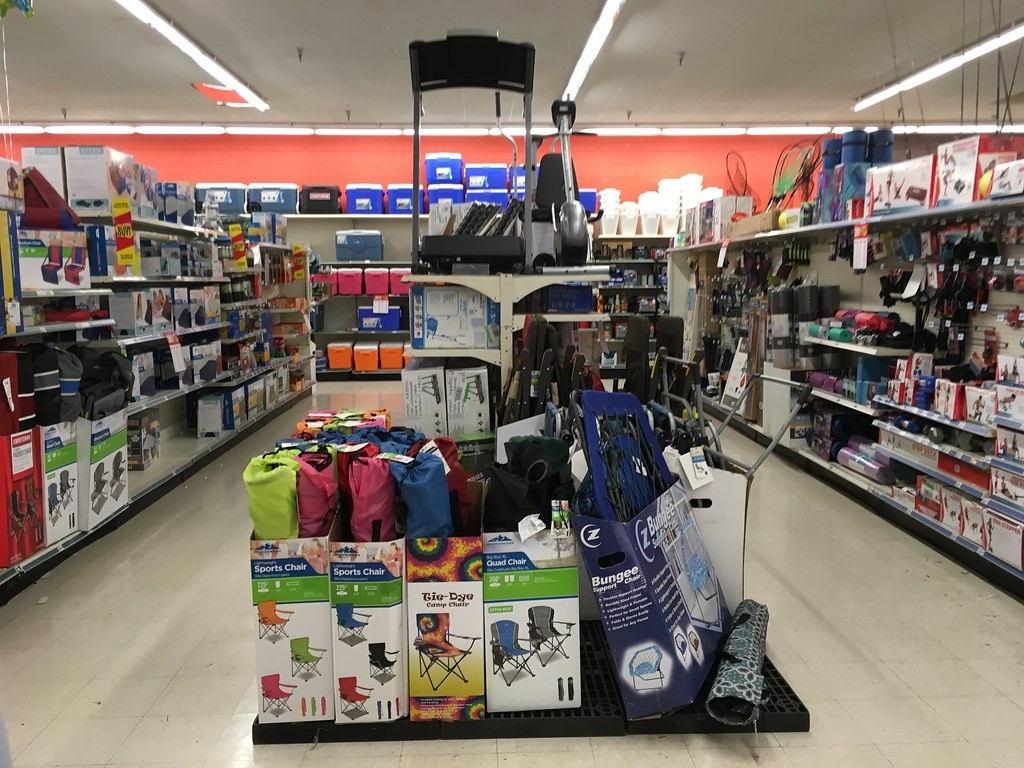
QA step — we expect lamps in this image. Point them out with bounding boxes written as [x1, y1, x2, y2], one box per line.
[853, 18, 1024, 112]
[0, 122, 1024, 137]
[112, 0, 272, 112]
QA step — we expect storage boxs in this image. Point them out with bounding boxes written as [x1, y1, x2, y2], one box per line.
[921, 231, 936, 256]
[18, 229, 91, 291]
[685, 196, 753, 247]
[250, 474, 581, 723]
[251, 212, 287, 244]
[290, 369, 304, 391]
[22, 147, 195, 226]
[615, 323, 628, 338]
[887, 352, 1024, 430]
[23, 304, 46, 327]
[195, 153, 597, 214]
[540, 281, 591, 313]
[314, 268, 416, 295]
[410, 286, 500, 350]
[358, 305, 402, 330]
[846, 197, 865, 221]
[229, 310, 246, 339]
[699, 251, 721, 378]
[570, 459, 755, 721]
[266, 297, 308, 308]
[990, 426, 1024, 510]
[604, 241, 634, 260]
[596, 173, 723, 235]
[79, 223, 222, 277]
[720, 337, 749, 416]
[403, 357, 495, 473]
[124, 338, 222, 402]
[864, 135, 1024, 218]
[731, 208, 780, 238]
[879, 427, 990, 490]
[186, 363, 289, 439]
[109, 283, 222, 338]
[893, 475, 1024, 572]
[927, 263, 944, 288]
[273, 323, 309, 334]
[327, 339, 415, 372]
[336, 230, 385, 261]
[601, 352, 617, 367]
[811, 408, 860, 462]
[311, 302, 324, 332]
[763, 360, 858, 449]
[128, 408, 161, 470]
[744, 297, 768, 427]
[254, 342, 271, 368]
[44, 295, 101, 343]
[1, 406, 128, 567]
[421, 235, 522, 269]
[603, 264, 654, 286]
[843, 378, 856, 402]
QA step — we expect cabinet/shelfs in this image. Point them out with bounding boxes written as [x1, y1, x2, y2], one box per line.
[669, 199, 1024, 601]
[593, 235, 678, 379]
[197, 214, 429, 380]
[401, 273, 613, 423]
[0, 209, 316, 606]
